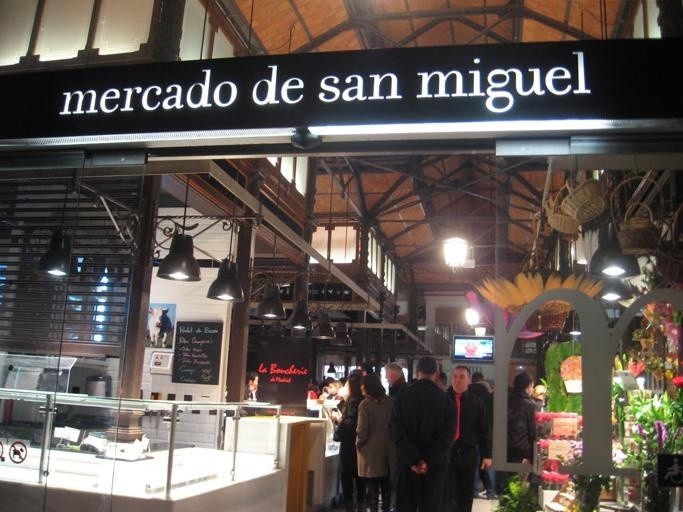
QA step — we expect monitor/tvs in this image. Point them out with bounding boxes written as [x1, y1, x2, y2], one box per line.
[453, 335, 496, 361]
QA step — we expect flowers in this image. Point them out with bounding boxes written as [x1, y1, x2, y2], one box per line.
[532, 358, 682, 489]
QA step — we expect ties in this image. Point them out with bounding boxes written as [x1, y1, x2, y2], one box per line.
[453, 394, 460, 442]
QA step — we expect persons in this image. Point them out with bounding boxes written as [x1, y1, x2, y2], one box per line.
[306, 357, 550, 511]
[243, 372, 259, 416]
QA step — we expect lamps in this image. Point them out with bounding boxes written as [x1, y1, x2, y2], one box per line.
[40, 179, 79, 277]
[156, 175, 354, 347]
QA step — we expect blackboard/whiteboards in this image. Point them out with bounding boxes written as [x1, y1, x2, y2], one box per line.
[172, 321, 223, 385]
